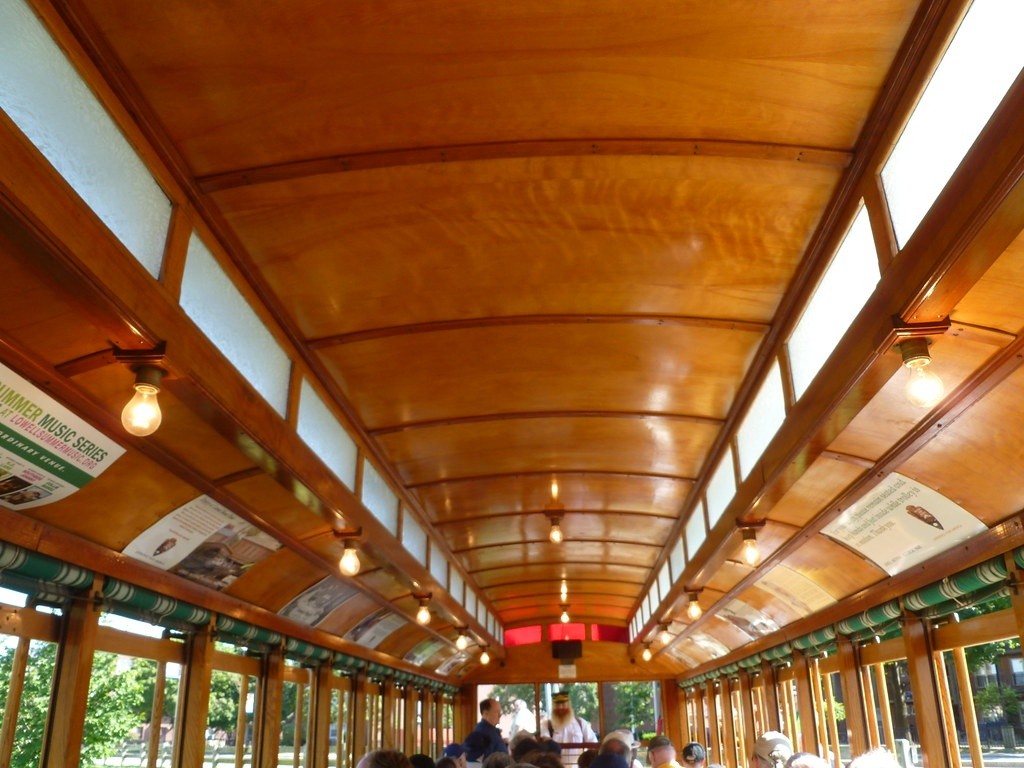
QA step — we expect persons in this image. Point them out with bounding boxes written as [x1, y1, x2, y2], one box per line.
[459, 698, 509, 757]
[540, 691, 599, 768]
[359, 729, 898, 768]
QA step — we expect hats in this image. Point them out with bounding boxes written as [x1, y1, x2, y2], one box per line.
[552, 691, 570, 703]
[509, 730, 537, 750]
[615, 729, 641, 748]
[755, 731, 794, 765]
[646, 736, 673, 764]
[442, 743, 470, 760]
[682, 743, 706, 764]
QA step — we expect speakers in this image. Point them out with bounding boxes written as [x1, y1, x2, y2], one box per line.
[553, 640, 582, 659]
